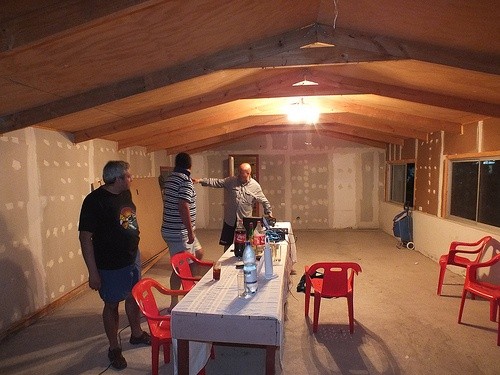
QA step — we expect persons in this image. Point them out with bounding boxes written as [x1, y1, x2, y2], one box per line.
[161, 153, 204, 308]
[191, 163, 273, 253]
[78, 161, 153, 369]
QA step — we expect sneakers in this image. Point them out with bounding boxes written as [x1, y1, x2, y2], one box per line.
[130, 330, 153, 345]
[108, 347, 127, 370]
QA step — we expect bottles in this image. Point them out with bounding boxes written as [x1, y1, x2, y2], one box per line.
[249, 221, 267, 261]
[242, 242, 258, 294]
[265, 243, 272, 277]
[234, 219, 246, 257]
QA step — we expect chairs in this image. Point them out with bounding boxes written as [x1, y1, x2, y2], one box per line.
[170, 250, 217, 290]
[131, 277, 216, 374]
[296, 261, 362, 335]
[457, 252, 500, 346]
[436, 235, 493, 300]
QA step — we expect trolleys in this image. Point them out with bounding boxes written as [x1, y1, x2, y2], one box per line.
[393, 201, 417, 251]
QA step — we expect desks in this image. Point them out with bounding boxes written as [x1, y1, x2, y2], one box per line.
[169, 221, 297, 375]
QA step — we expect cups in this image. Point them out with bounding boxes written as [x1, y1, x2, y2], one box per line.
[269, 241, 281, 262]
[213, 261, 221, 281]
[237, 274, 246, 297]
